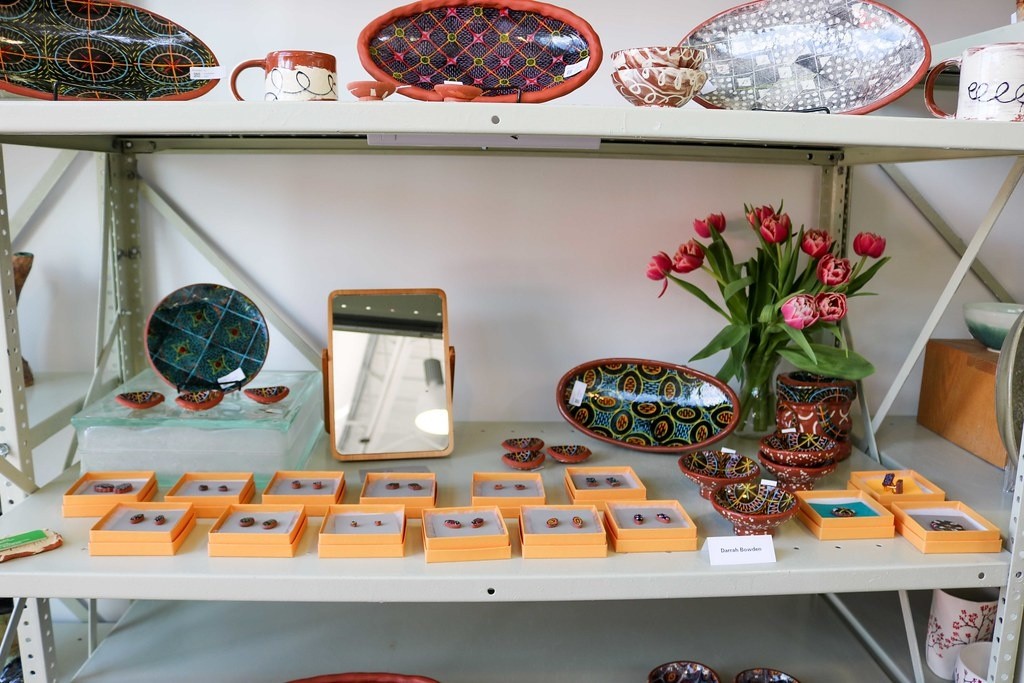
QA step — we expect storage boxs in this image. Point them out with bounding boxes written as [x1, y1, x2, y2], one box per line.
[262, 471, 345, 515]
[890, 501, 1002, 553]
[422, 505, 511, 562]
[88, 502, 196, 556]
[471, 470, 545, 517]
[847, 469, 945, 512]
[164, 473, 256, 517]
[318, 504, 407, 558]
[70, 367, 324, 489]
[605, 499, 697, 552]
[519, 505, 607, 557]
[63, 471, 159, 517]
[208, 504, 307, 558]
[795, 490, 895, 539]
[564, 466, 647, 511]
[360, 472, 438, 518]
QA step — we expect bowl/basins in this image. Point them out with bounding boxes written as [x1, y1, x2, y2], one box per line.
[962, 302, 1024, 354]
[675, 370, 857, 539]
[610, 47, 708, 109]
[434, 84, 481, 102]
[349, 82, 396, 101]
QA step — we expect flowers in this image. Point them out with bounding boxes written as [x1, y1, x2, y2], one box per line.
[647, 197, 891, 431]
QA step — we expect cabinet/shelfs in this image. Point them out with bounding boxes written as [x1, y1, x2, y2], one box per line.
[0, 0, 1024, 683]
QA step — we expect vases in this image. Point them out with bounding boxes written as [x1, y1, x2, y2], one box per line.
[733, 352, 782, 438]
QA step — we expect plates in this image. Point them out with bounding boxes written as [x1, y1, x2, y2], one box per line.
[51, 0, 221, 102]
[114, 282, 290, 408]
[678, 0, 933, 117]
[355, 0, 604, 104]
[555, 358, 742, 453]
[648, 661, 801, 682]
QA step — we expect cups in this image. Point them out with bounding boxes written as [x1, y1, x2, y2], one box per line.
[924, 43, 1024, 123]
[954, 642, 1024, 682]
[231, 48, 338, 102]
[925, 587, 998, 680]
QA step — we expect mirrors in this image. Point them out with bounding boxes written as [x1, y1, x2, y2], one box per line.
[322, 288, 455, 462]
